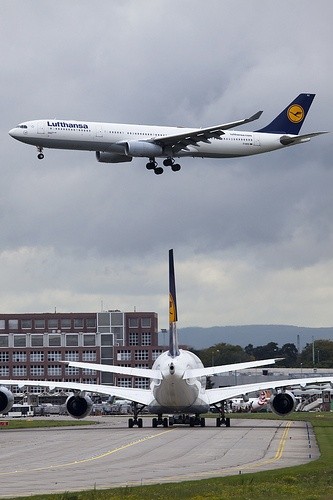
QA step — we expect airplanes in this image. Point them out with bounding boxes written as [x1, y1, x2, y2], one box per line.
[0, 249, 333, 428]
[9, 93, 327, 174]
